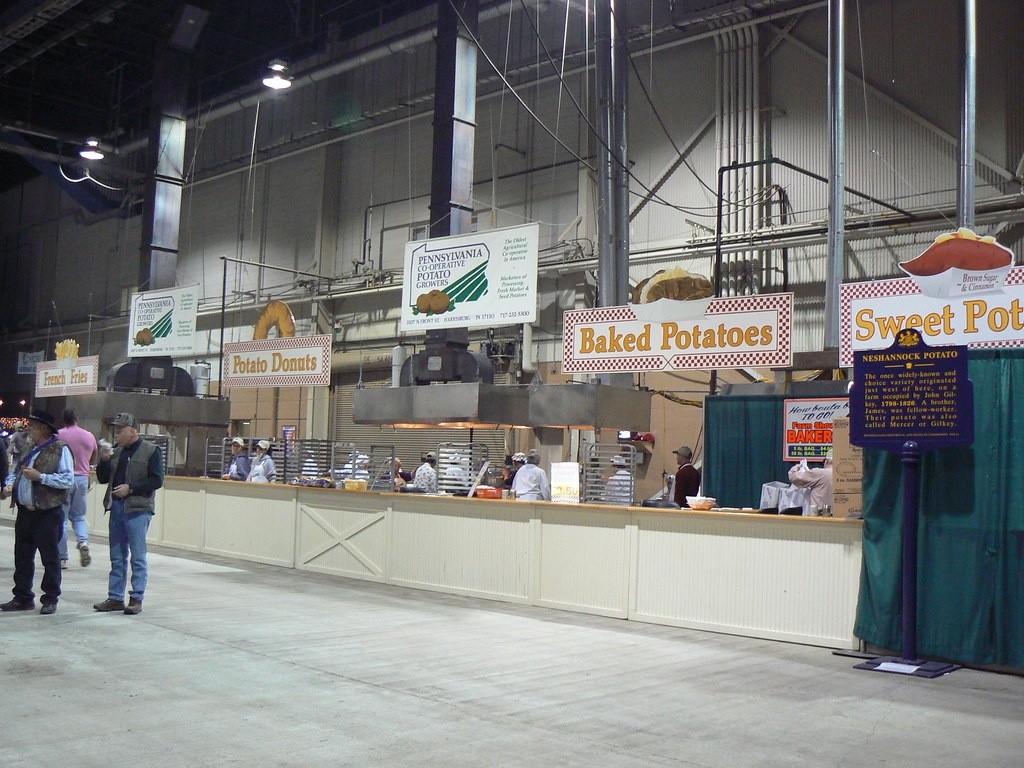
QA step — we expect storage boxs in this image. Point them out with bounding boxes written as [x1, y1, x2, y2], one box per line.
[475, 488, 503, 498]
[344, 480, 369, 490]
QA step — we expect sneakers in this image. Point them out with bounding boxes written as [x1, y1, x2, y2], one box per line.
[93, 598, 126, 612]
[124, 597, 142, 614]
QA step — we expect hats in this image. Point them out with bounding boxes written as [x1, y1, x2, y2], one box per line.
[256, 440, 270, 451]
[426, 452, 437, 460]
[27, 409, 58, 434]
[610, 456, 627, 466]
[672, 446, 692, 458]
[111, 412, 138, 429]
[511, 452, 527, 463]
[231, 437, 244, 448]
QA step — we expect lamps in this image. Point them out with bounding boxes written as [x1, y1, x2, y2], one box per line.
[78, 135, 106, 160]
[261, 59, 292, 89]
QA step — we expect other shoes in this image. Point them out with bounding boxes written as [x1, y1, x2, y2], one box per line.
[61, 559, 69, 569]
[0, 598, 36, 611]
[40, 603, 58, 614]
[79, 542, 91, 567]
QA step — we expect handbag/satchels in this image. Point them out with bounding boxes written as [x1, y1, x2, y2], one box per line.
[9, 445, 19, 454]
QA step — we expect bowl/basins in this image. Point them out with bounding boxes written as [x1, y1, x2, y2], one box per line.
[686, 496, 717, 511]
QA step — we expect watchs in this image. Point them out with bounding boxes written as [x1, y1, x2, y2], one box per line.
[128, 483, 133, 496]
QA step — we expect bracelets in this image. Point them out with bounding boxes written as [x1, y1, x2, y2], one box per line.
[39, 474, 43, 482]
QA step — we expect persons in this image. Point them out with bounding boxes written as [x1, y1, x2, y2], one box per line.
[53, 406, 98, 569]
[788, 448, 833, 516]
[605, 455, 635, 505]
[222, 437, 250, 480]
[379, 457, 418, 491]
[0, 409, 74, 614]
[513, 449, 551, 501]
[93, 413, 164, 614]
[445, 453, 469, 493]
[245, 440, 277, 484]
[301, 458, 322, 477]
[413, 452, 438, 493]
[502, 452, 526, 490]
[673, 446, 701, 508]
[344, 453, 371, 480]
